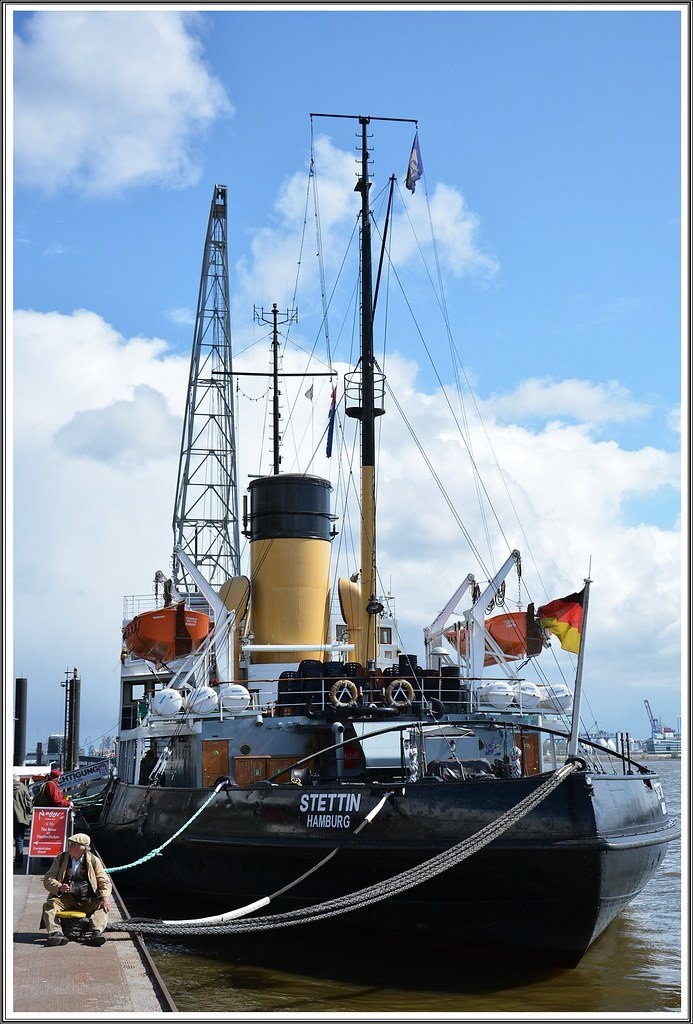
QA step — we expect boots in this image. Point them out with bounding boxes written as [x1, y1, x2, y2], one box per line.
[47, 933, 69, 946]
[84, 932, 106, 947]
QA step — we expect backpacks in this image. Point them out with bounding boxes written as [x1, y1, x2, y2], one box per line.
[32, 780, 56, 807]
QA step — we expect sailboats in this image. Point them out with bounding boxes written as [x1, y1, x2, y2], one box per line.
[71, 107, 680, 995]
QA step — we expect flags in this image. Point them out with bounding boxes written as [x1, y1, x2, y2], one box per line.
[535, 585, 584, 655]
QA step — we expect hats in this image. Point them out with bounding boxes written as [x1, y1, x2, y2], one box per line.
[51, 769, 61, 777]
[68, 833, 90, 845]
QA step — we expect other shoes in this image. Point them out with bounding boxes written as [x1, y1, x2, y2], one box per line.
[13, 861, 22, 868]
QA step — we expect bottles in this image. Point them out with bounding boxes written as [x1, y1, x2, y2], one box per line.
[65, 877, 71, 891]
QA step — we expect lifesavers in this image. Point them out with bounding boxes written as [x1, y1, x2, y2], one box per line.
[428, 698, 445, 720]
[386, 679, 415, 708]
[331, 679, 358, 707]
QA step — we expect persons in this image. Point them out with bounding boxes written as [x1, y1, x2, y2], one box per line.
[39, 834, 112, 947]
[13, 773, 32, 864]
[40, 769, 75, 809]
